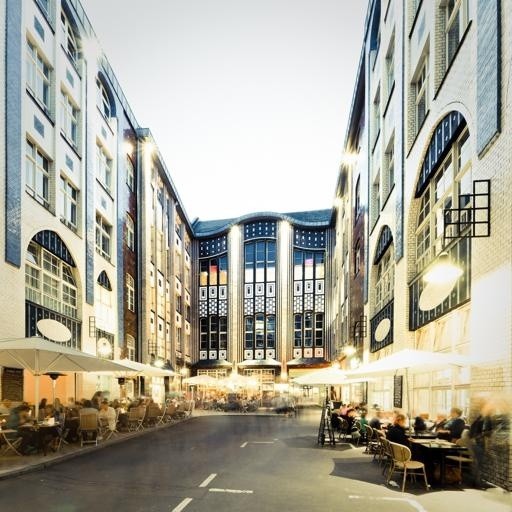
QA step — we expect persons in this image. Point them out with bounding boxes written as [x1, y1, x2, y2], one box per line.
[329, 386, 494, 489]
[0, 390, 297, 458]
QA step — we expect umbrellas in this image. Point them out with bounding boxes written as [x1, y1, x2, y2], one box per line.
[292, 349, 459, 437]
[0, 338, 265, 416]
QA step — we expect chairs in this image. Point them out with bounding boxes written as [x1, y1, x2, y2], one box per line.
[0, 397, 194, 456]
[335, 413, 479, 496]
